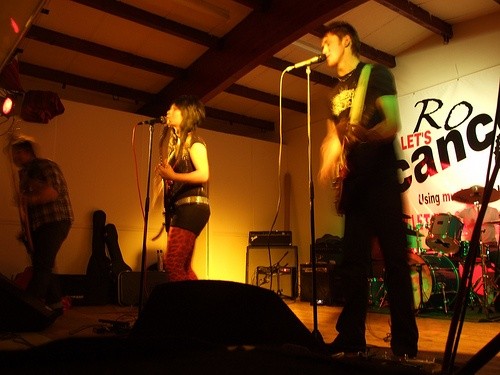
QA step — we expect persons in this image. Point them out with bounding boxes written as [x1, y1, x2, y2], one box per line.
[316, 21, 420, 358]
[155, 94, 211, 281]
[11, 138, 75, 316]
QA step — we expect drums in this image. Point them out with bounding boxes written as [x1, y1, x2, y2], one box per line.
[457, 241, 488, 264]
[408, 248, 459, 311]
[426, 213, 464, 253]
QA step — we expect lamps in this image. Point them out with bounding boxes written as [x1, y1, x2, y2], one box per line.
[0, 92, 24, 120]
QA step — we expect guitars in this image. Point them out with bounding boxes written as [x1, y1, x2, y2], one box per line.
[158, 124, 170, 232]
[334, 62, 371, 215]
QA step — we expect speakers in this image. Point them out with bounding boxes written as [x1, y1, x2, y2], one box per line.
[132, 279, 322, 354]
[0, 272, 56, 332]
[246, 245, 299, 299]
[300, 264, 332, 303]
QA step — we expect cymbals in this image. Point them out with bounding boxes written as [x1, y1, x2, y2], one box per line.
[406, 227, 424, 236]
[453, 185, 500, 205]
[403, 213, 412, 220]
[484, 220, 500, 226]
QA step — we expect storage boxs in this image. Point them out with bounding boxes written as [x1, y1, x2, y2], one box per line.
[244, 244, 301, 301]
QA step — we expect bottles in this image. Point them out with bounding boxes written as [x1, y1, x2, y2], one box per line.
[156, 250, 164, 272]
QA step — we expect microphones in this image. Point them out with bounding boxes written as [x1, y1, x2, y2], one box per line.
[285, 54, 326, 72]
[138, 116, 166, 125]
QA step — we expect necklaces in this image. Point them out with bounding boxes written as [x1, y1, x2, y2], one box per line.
[337, 72, 354, 82]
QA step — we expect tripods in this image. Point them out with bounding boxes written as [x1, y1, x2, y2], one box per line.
[468, 244, 500, 314]
